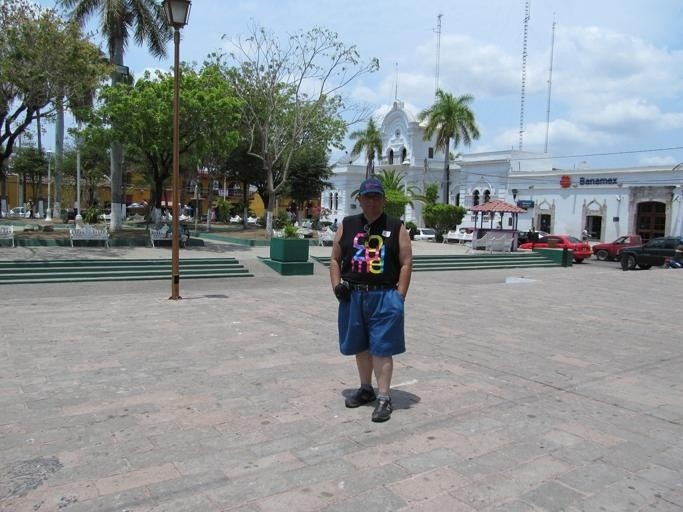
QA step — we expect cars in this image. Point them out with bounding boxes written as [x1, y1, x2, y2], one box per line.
[408, 225, 682, 272]
[9, 207, 30, 218]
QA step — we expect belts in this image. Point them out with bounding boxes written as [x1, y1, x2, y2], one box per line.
[342, 280, 394, 293]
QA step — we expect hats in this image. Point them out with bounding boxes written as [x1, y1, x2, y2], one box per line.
[358, 178, 386, 198]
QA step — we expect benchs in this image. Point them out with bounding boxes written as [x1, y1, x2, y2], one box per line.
[149, 223, 192, 250]
[69, 224, 111, 248]
[230, 215, 242, 223]
[0, 224, 15, 248]
[316, 226, 337, 248]
[247, 216, 257, 224]
[442, 230, 474, 245]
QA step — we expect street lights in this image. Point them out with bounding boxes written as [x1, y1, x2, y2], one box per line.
[155, 0, 196, 301]
[44, 146, 54, 220]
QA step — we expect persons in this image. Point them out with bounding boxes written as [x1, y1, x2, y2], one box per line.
[26, 199, 34, 219]
[330, 178, 412, 422]
[583, 226, 591, 244]
[286, 202, 321, 227]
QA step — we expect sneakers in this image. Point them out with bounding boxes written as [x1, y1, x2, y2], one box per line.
[344, 389, 376, 408]
[370, 399, 393, 423]
